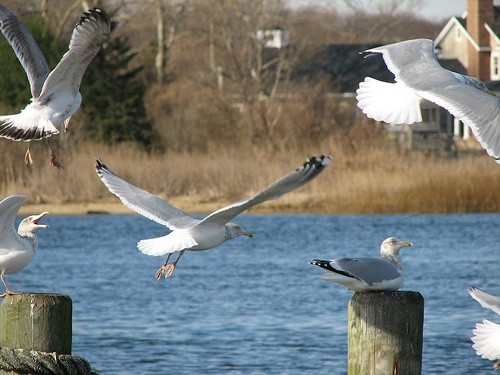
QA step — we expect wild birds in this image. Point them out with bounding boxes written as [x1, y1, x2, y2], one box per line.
[0, 195, 49, 298]
[307, 238, 414, 290]
[0, 6, 112, 170]
[93, 154, 333, 281]
[468, 284, 500, 370]
[355, 39, 500, 164]
